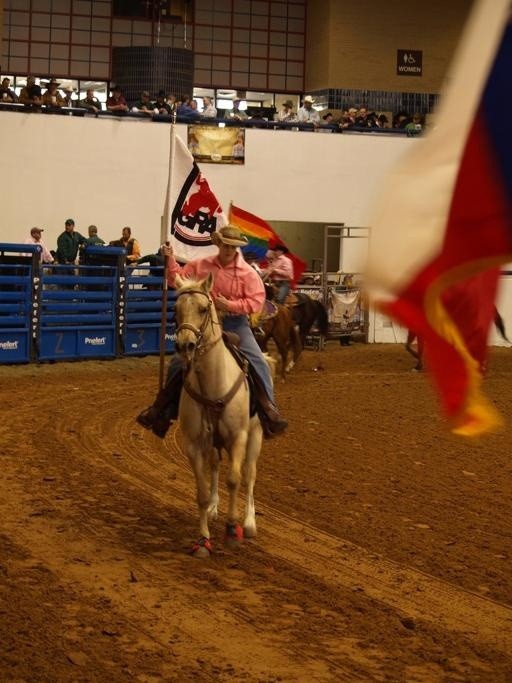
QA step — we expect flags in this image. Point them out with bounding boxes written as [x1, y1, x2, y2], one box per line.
[228, 204, 306, 291]
[167, 133, 245, 265]
[357, 1, 512, 438]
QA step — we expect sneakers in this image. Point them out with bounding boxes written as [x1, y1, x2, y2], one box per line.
[342, 342, 351, 345]
[139, 406, 172, 438]
[261, 417, 289, 440]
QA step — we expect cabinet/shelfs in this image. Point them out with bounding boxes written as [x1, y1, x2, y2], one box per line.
[289, 224, 370, 352]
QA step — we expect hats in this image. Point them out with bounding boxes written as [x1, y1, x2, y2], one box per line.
[282, 100, 293, 108]
[31, 227, 43, 234]
[300, 95, 316, 103]
[232, 97, 240, 102]
[348, 108, 357, 113]
[272, 243, 288, 253]
[65, 219, 74, 225]
[46, 79, 73, 92]
[156, 90, 167, 98]
[109, 85, 124, 91]
[244, 252, 259, 259]
[367, 112, 388, 122]
[211, 226, 248, 248]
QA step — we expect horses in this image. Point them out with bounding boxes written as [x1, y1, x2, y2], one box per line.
[175, 271, 275, 559]
[252, 303, 302, 384]
[264, 286, 328, 336]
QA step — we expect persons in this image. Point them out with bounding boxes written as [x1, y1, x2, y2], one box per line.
[21, 219, 165, 292]
[250, 243, 294, 305]
[136, 225, 288, 438]
[303, 274, 354, 347]
